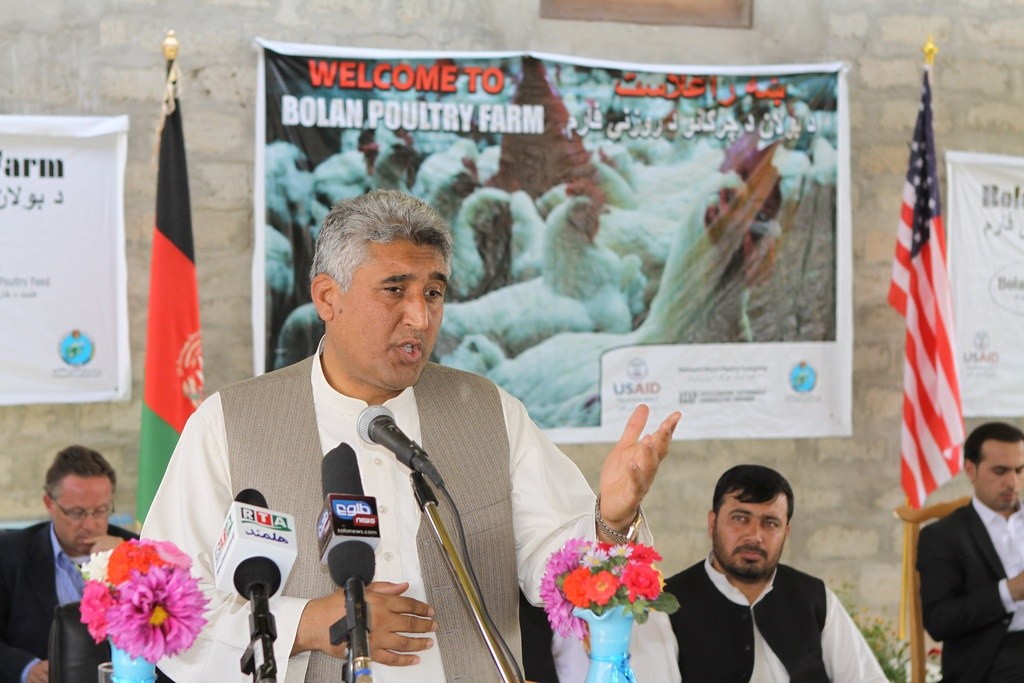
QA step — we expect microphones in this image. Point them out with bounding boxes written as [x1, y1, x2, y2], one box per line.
[213, 488, 298, 683]
[317, 442, 372, 683]
[357, 406, 445, 489]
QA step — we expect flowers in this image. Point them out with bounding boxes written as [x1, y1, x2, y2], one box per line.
[539, 535, 681, 641]
[74, 537, 215, 664]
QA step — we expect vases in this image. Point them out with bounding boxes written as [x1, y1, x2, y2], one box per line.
[106, 635, 158, 683]
[572, 603, 650, 683]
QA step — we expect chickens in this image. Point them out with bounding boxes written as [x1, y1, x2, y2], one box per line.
[265, 59, 836, 426]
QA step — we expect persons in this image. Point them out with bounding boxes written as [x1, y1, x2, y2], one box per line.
[915, 422, 1024, 683]
[663, 464, 890, 683]
[0, 445, 140, 683]
[140, 187, 683, 683]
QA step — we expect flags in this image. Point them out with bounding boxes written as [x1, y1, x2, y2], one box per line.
[886, 64, 967, 509]
[133, 61, 205, 528]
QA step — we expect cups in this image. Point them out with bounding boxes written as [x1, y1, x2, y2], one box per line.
[98, 662, 113, 683]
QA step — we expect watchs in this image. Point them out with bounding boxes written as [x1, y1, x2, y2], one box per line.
[595, 493, 644, 545]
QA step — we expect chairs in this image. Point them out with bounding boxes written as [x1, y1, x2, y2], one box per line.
[895, 495, 973, 683]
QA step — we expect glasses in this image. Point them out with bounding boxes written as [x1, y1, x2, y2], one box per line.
[51, 496, 117, 519]
